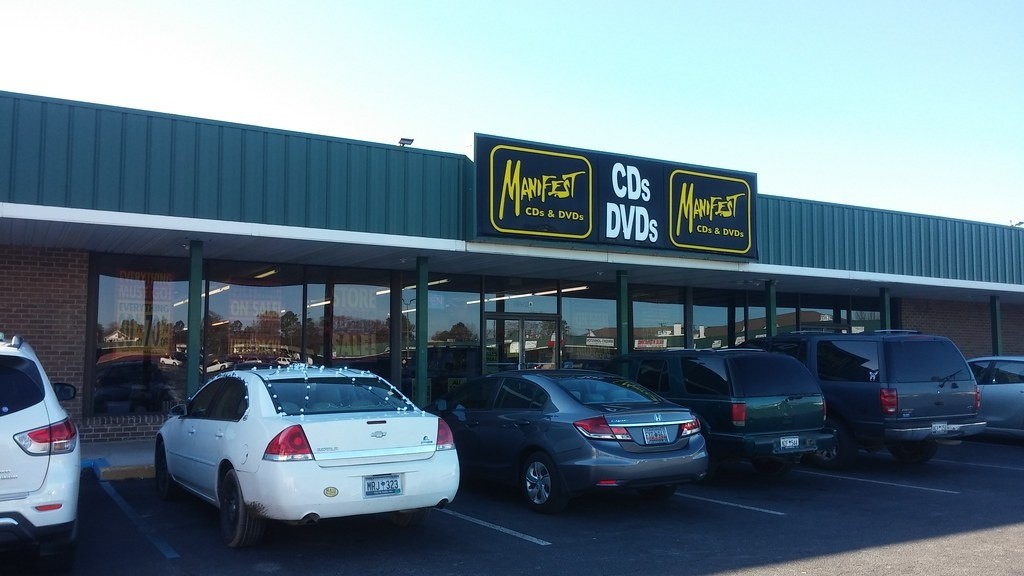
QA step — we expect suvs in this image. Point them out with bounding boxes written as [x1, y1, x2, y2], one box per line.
[0, 332, 79, 551]
[739, 329, 981, 463]
[612, 346, 838, 477]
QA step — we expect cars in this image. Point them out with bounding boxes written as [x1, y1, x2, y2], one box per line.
[245, 357, 263, 364]
[199, 358, 230, 374]
[275, 357, 292, 365]
[966, 355, 1024, 443]
[160, 356, 182, 366]
[435, 373, 708, 516]
[154, 363, 458, 551]
[93, 350, 220, 413]
